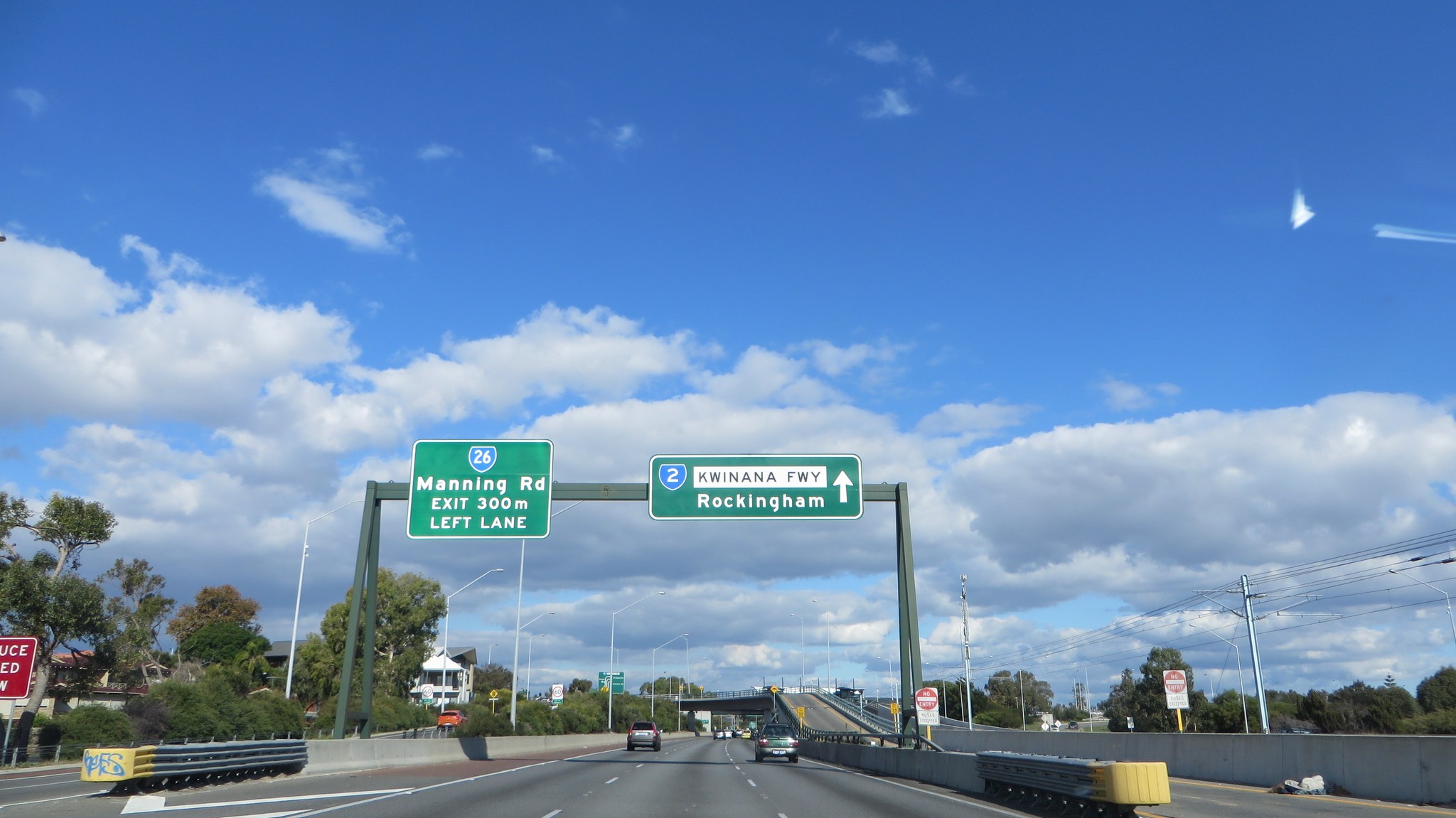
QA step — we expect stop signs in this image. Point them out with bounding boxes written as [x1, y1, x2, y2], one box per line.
[915, 687, 939, 712]
[1162, 670, 1188, 694]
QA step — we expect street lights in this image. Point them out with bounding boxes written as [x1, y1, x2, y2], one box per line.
[510, 503, 584, 732]
[682, 676, 690, 694]
[679, 636, 690, 695]
[1189, 624, 1249, 733]
[791, 613, 806, 693]
[925, 663, 948, 718]
[866, 669, 879, 704]
[1072, 663, 1098, 733]
[610, 647, 620, 671]
[526, 634, 546, 704]
[700, 675, 722, 697]
[607, 591, 666, 735]
[488, 643, 499, 666]
[1388, 569, 1455, 643]
[876, 683, 899, 733]
[935, 673, 964, 722]
[877, 656, 894, 701]
[664, 671, 671, 699]
[990, 654, 1050, 731]
[813, 600, 830, 695]
[442, 567, 506, 716]
[650, 633, 692, 717]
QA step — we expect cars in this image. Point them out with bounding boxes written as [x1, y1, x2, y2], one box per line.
[742, 730, 752, 740]
[438, 711, 468, 730]
[713, 729, 737, 740]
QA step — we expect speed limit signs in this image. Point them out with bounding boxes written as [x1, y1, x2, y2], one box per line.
[422, 684, 434, 700]
[552, 684, 564, 700]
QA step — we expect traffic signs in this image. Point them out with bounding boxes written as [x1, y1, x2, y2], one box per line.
[647, 453, 865, 522]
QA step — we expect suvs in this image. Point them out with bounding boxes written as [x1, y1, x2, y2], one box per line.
[626, 721, 663, 751]
[754, 723, 799, 764]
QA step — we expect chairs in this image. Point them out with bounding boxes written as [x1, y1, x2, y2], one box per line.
[766, 731, 789, 736]
